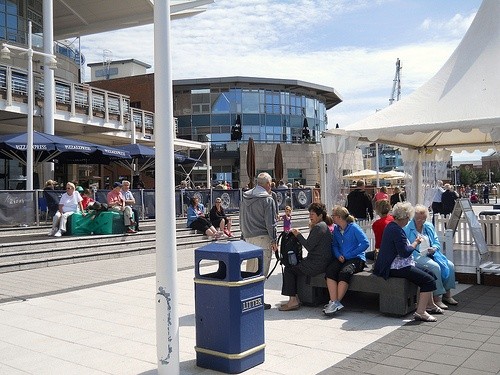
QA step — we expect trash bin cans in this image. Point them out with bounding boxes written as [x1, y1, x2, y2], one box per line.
[192, 238, 267, 374]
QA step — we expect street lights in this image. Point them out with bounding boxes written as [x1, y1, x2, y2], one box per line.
[1, 20, 58, 191]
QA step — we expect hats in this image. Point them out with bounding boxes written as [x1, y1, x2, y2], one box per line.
[113, 182, 124, 187]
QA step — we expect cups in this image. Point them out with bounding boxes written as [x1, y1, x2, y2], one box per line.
[195, 230, 197, 234]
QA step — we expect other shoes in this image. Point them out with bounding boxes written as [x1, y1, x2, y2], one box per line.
[444, 298, 458, 304]
[130, 221, 137, 226]
[126, 229, 137, 233]
[436, 302, 448, 309]
[278, 302, 302, 310]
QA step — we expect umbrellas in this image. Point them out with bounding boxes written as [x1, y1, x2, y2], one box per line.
[110, 143, 202, 189]
[0, 130, 132, 173]
[342, 168, 413, 186]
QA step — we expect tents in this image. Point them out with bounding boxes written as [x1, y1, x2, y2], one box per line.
[318, 0, 500, 215]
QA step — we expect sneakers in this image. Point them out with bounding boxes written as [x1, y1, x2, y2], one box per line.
[54, 229, 63, 237]
[48, 230, 57, 236]
[322, 300, 344, 313]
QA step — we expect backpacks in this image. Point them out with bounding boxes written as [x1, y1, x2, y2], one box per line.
[275, 231, 303, 266]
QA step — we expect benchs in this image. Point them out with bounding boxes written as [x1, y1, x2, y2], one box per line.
[67, 210, 131, 234]
[299, 259, 421, 317]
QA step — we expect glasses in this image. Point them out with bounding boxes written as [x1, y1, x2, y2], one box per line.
[331, 215, 340, 219]
[65, 187, 72, 190]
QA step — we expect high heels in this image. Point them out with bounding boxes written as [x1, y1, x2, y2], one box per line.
[426, 306, 444, 313]
[413, 311, 437, 322]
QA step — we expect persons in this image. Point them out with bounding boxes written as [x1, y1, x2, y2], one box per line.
[278, 206, 292, 231]
[457, 184, 489, 204]
[47, 182, 86, 236]
[428, 180, 459, 229]
[81, 180, 143, 233]
[298, 137, 302, 143]
[345, 178, 407, 221]
[186, 195, 224, 238]
[209, 197, 236, 238]
[311, 138, 316, 144]
[304, 138, 308, 143]
[176, 180, 233, 194]
[240, 173, 278, 310]
[244, 180, 304, 189]
[42, 180, 84, 218]
[323, 207, 369, 316]
[315, 182, 320, 188]
[278, 202, 334, 311]
[135, 182, 147, 189]
[365, 199, 458, 322]
